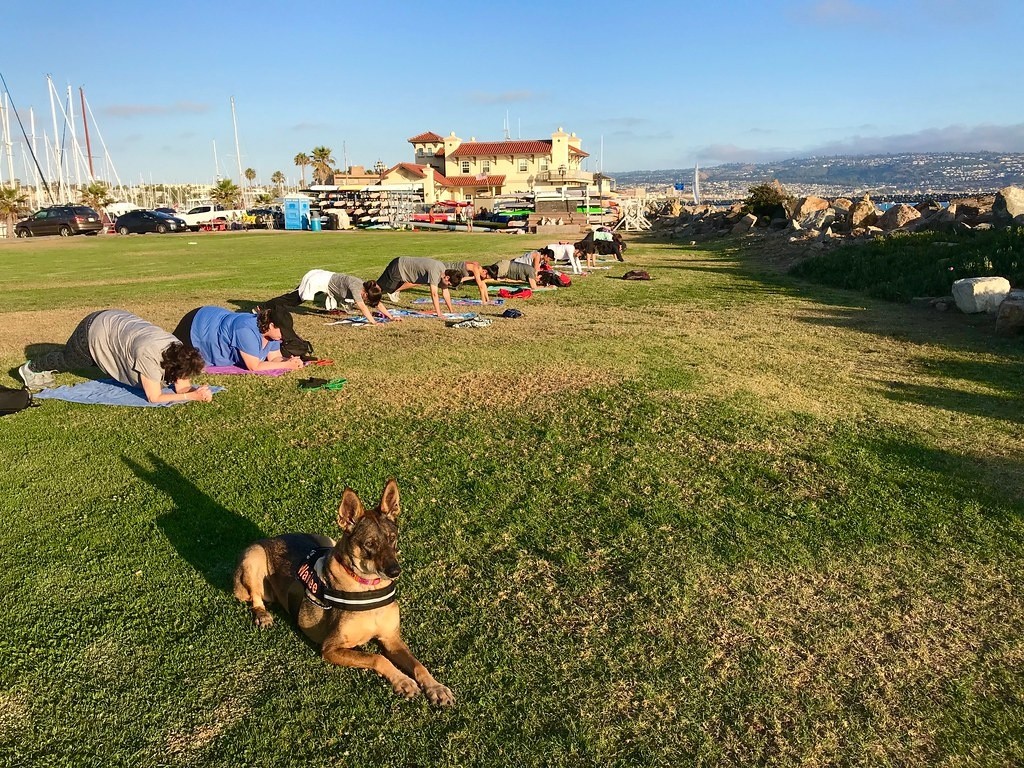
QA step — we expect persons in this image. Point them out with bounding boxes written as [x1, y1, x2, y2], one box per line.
[376, 255, 465, 320]
[493, 259, 558, 290]
[512, 248, 555, 273]
[172, 306, 305, 370]
[444, 261, 499, 304]
[454, 203, 463, 225]
[544, 227, 626, 275]
[464, 202, 475, 232]
[282, 269, 403, 326]
[429, 205, 436, 230]
[18, 309, 213, 403]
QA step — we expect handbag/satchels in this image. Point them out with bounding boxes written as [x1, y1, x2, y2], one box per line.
[557, 273, 570, 287]
[282, 337, 313, 357]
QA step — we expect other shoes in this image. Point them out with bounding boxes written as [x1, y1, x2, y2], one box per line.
[251, 306, 262, 314]
[19, 359, 44, 390]
[341, 303, 356, 314]
[41, 370, 59, 387]
[386, 291, 400, 303]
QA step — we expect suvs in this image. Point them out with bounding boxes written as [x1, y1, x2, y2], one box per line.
[13, 203, 104, 239]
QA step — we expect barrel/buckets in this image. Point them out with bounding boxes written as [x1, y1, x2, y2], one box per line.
[310, 216, 321, 231]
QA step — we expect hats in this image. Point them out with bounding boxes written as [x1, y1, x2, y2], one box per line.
[269, 311, 298, 340]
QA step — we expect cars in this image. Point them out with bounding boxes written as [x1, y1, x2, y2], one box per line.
[114, 209, 188, 235]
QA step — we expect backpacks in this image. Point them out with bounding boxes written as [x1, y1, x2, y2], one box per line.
[623, 270, 650, 280]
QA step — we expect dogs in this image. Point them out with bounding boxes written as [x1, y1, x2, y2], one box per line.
[229, 477, 458, 709]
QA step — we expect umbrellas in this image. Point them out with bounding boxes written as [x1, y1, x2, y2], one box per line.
[437, 199, 473, 208]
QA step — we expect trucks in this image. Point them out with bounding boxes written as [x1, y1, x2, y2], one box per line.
[174, 204, 248, 232]
